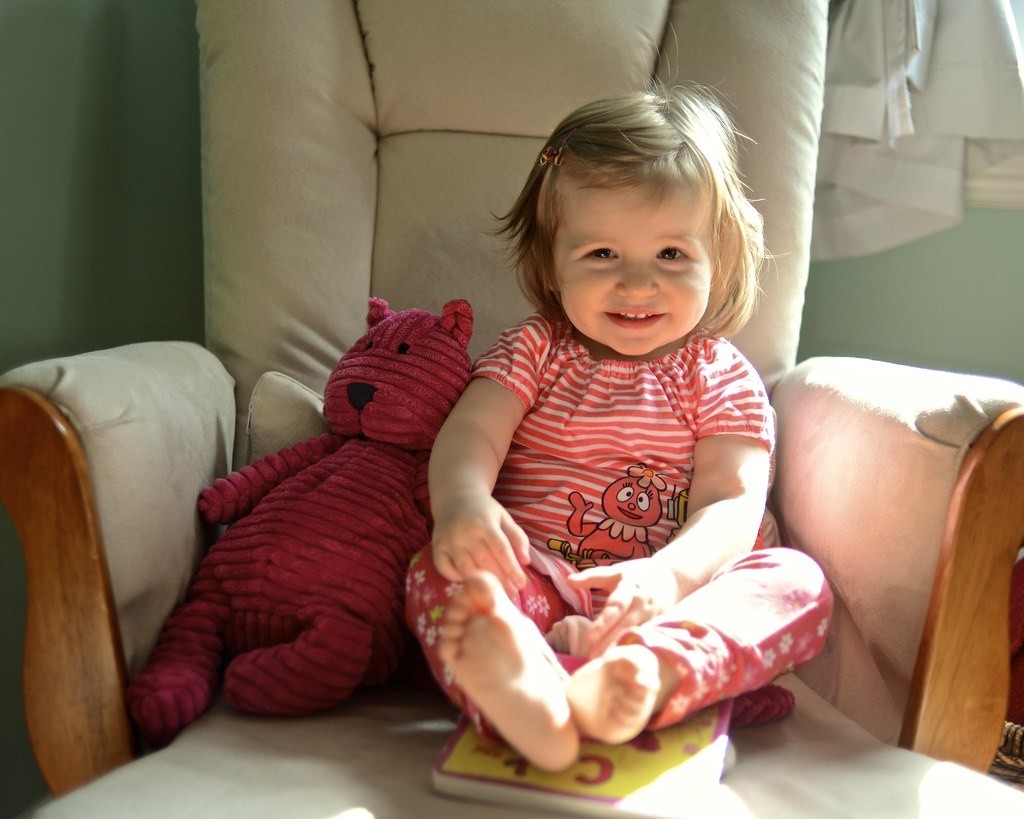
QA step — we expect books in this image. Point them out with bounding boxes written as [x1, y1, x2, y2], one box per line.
[430, 697, 733, 819]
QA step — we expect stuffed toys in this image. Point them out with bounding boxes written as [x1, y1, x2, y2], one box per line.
[125, 298, 475, 737]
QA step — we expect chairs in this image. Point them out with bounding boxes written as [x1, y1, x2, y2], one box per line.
[0, 0, 1024, 819]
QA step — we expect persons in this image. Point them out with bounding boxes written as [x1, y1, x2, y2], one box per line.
[406, 89, 834, 773]
